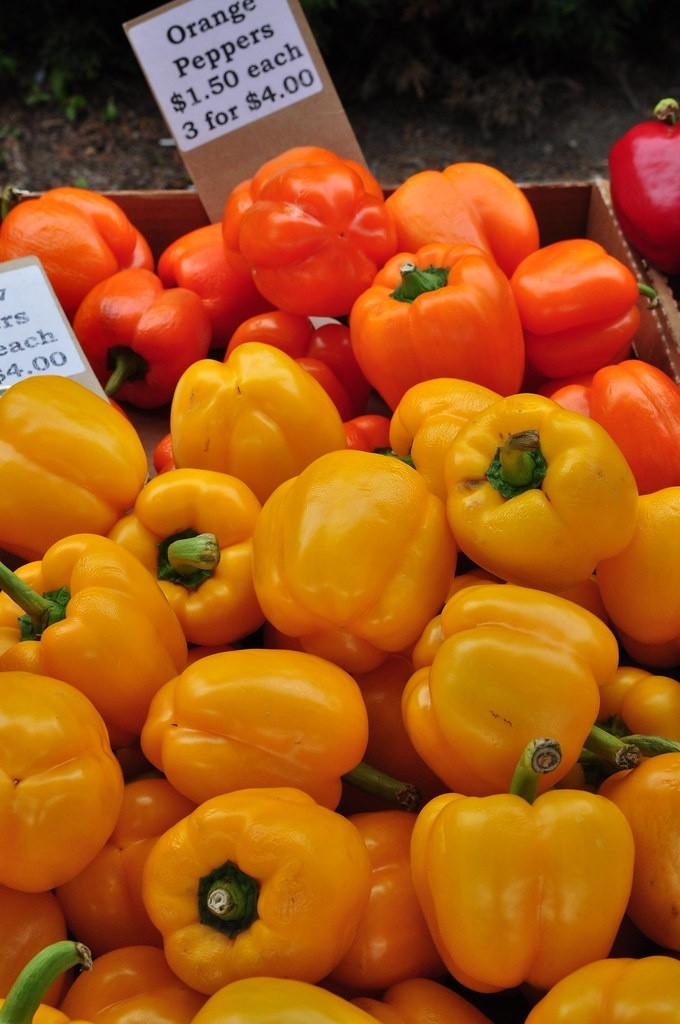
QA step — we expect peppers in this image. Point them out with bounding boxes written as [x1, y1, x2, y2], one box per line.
[0, 95, 680, 1024]
